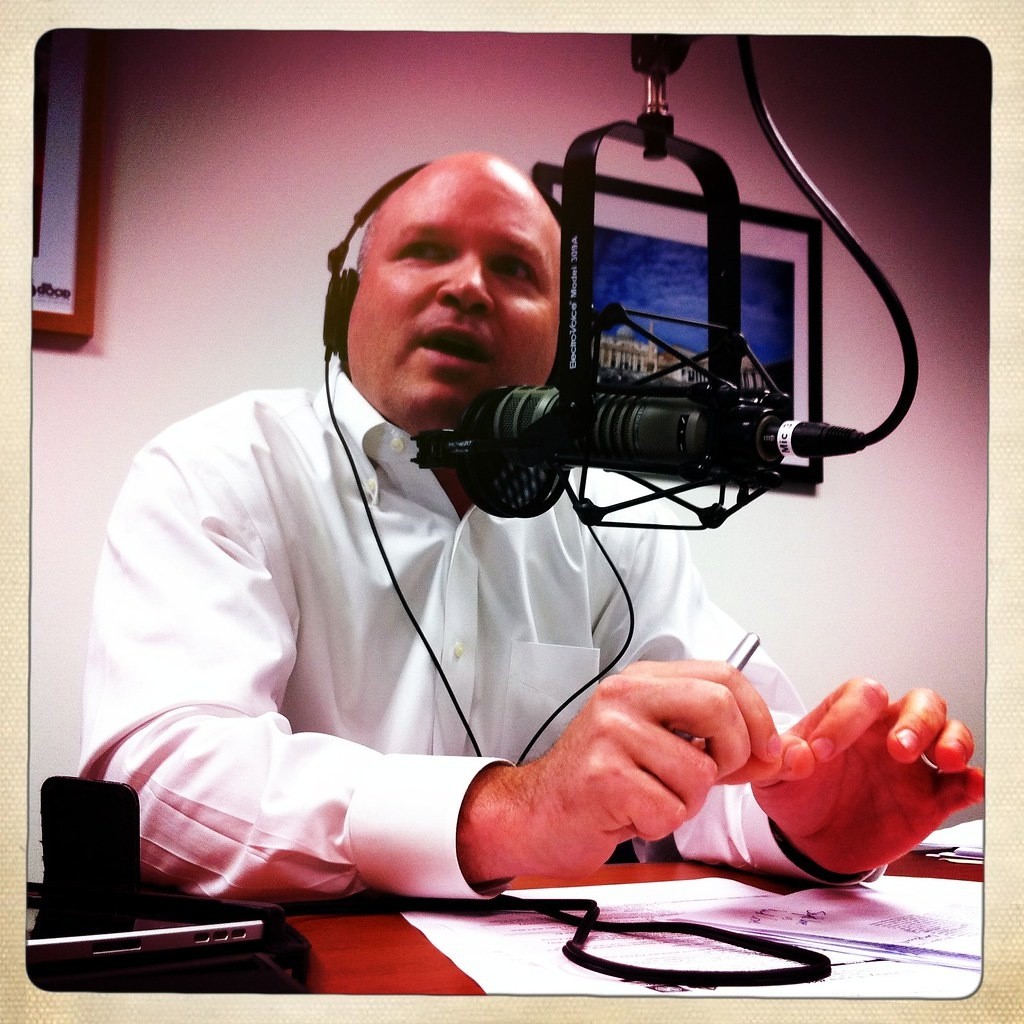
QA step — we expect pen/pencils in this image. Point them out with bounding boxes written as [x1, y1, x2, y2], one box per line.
[671, 630, 763, 744]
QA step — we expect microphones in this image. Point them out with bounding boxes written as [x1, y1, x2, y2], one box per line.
[495, 384, 862, 478]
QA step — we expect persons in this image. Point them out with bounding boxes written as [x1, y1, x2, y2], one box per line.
[78, 153, 986, 906]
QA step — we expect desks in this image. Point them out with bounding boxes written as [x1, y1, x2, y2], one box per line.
[75, 846, 986, 999]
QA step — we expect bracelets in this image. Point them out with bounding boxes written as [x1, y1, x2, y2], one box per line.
[767, 816, 872, 883]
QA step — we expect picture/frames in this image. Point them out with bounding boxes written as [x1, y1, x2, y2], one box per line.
[31, 30, 123, 349]
[531, 160, 825, 488]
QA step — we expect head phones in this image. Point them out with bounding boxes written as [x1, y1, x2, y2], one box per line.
[325, 162, 562, 369]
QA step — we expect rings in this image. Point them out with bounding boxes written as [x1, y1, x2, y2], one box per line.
[919, 753, 939, 770]
